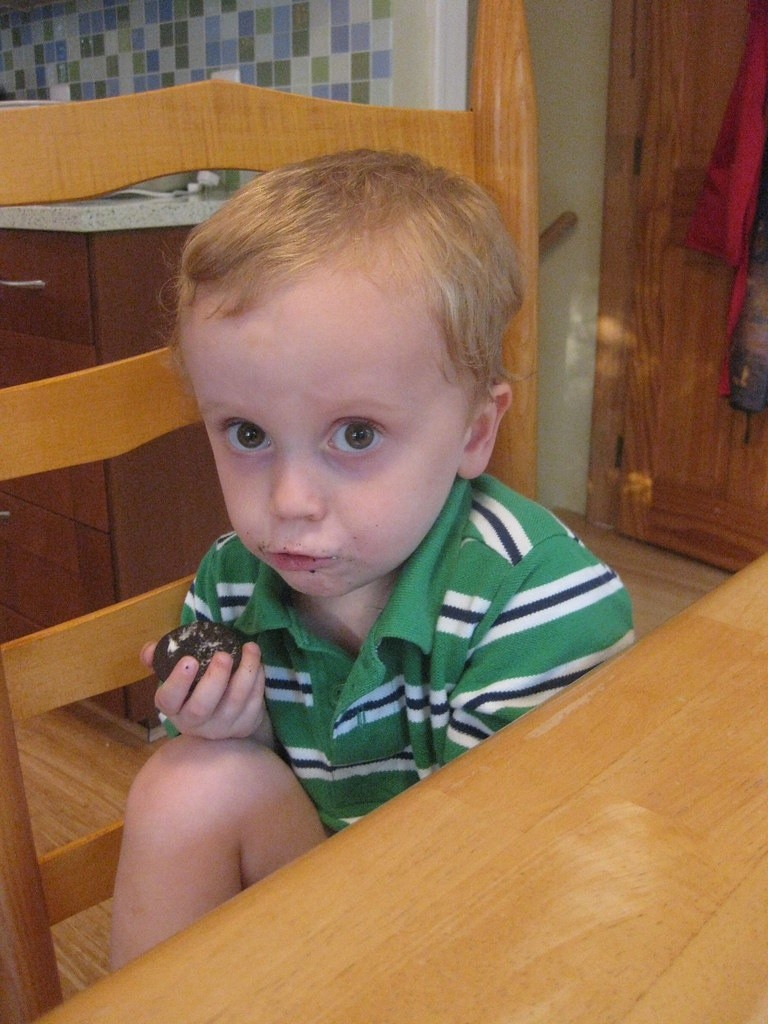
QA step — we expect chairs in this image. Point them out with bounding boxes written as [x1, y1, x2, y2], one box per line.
[0, 1, 540, 1024]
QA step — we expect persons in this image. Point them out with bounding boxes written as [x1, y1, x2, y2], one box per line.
[109, 149, 634, 972]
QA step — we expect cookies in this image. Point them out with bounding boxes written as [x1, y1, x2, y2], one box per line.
[152, 622, 248, 689]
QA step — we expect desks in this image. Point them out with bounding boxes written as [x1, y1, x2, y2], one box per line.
[43, 544, 767, 1024]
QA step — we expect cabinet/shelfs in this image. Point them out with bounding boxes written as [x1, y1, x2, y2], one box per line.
[2, 226, 237, 743]
